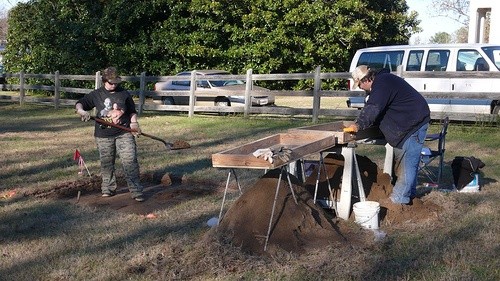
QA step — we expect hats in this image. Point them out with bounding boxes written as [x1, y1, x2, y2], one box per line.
[352, 65, 371, 90]
[102, 67, 121, 83]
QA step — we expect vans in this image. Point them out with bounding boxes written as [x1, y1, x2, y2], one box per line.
[346, 42, 500, 117]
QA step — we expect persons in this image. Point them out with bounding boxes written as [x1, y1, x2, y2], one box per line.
[75, 66, 146, 202]
[344, 65, 432, 205]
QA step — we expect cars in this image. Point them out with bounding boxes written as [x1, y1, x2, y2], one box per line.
[154, 70, 275, 113]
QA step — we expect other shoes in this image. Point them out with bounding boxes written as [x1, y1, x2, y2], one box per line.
[102, 193, 113, 197]
[134, 195, 144, 202]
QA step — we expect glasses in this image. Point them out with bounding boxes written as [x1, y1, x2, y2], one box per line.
[106, 80, 118, 85]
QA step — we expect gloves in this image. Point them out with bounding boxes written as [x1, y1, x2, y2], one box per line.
[271, 146, 293, 163]
[252, 147, 274, 164]
[343, 124, 360, 133]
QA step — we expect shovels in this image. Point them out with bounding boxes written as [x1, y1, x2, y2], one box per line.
[75, 109, 191, 150]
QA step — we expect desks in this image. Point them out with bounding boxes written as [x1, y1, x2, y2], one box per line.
[289, 121, 378, 207]
[212, 133, 338, 253]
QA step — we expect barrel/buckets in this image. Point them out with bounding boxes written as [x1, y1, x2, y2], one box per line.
[454, 174, 480, 193]
[352, 201, 381, 230]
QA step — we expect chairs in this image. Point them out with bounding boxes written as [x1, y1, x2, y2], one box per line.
[396, 117, 448, 187]
[475, 57, 488, 70]
